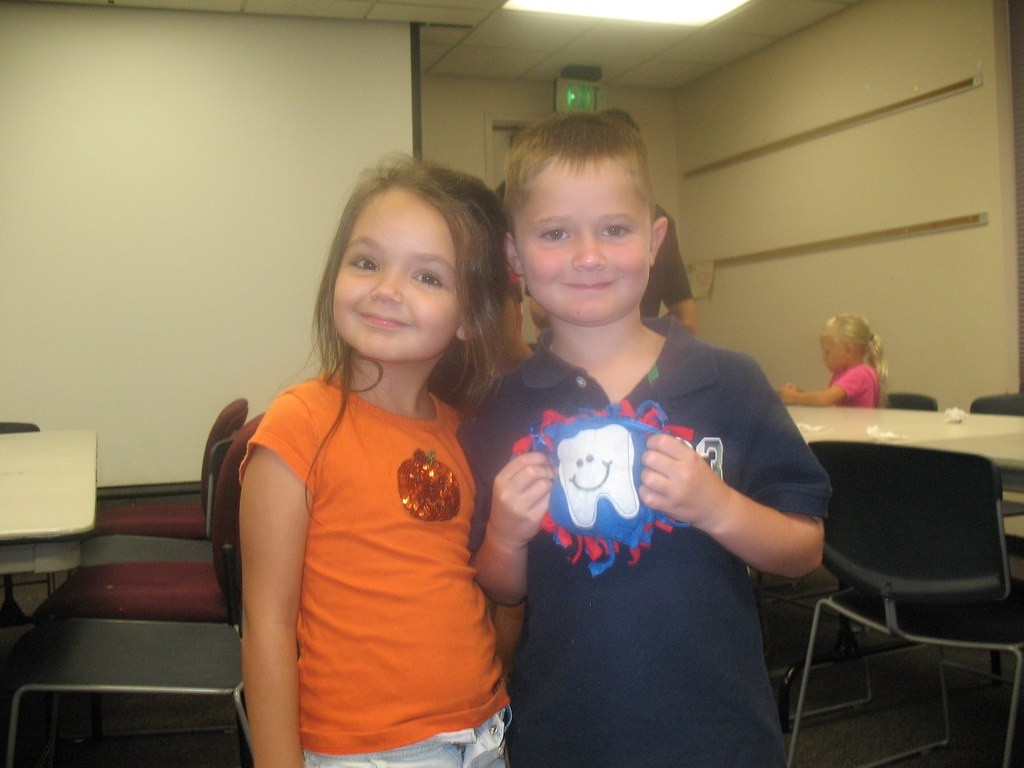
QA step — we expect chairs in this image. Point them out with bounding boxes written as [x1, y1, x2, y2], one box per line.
[886, 392, 939, 412]
[971, 394, 1024, 415]
[782, 437, 1024, 768]
[0, 398, 262, 768]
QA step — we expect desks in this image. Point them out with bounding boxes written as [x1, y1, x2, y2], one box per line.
[775, 405, 1024, 729]
[1, 431, 99, 625]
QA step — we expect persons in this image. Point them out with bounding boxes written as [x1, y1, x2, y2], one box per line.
[237, 158, 523, 768]
[458, 107, 831, 768]
[779, 315, 889, 408]
